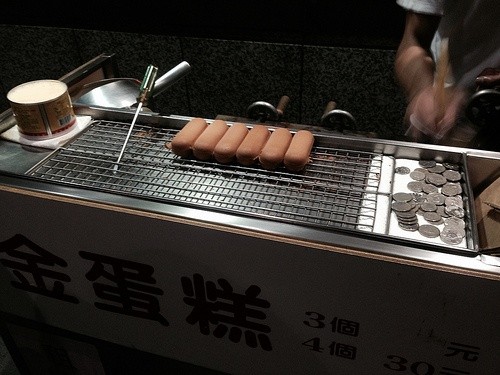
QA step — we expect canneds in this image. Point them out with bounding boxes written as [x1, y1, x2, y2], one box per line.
[6, 80, 76, 142]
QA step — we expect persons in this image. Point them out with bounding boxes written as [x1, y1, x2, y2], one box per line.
[395, 0, 500, 147]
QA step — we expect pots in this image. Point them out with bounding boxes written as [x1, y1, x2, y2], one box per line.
[68, 59, 191, 107]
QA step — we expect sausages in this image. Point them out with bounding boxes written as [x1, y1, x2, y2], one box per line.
[171, 118, 316, 169]
